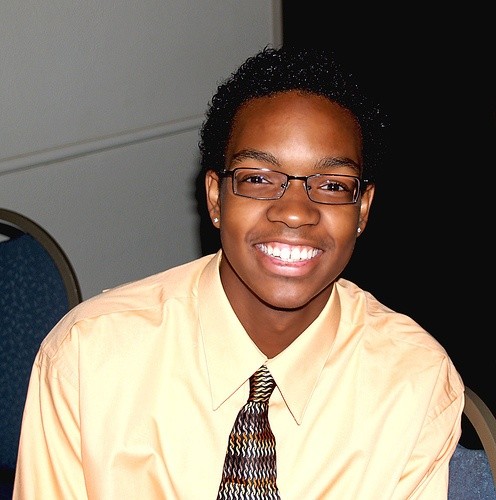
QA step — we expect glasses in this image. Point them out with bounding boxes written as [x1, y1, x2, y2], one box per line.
[219, 167, 369, 205]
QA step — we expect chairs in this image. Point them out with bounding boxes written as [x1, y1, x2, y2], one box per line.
[0, 207, 86, 499]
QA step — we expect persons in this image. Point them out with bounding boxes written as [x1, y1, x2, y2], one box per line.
[9, 45, 466, 499]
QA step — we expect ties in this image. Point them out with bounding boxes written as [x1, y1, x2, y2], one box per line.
[216, 365, 283, 500]
